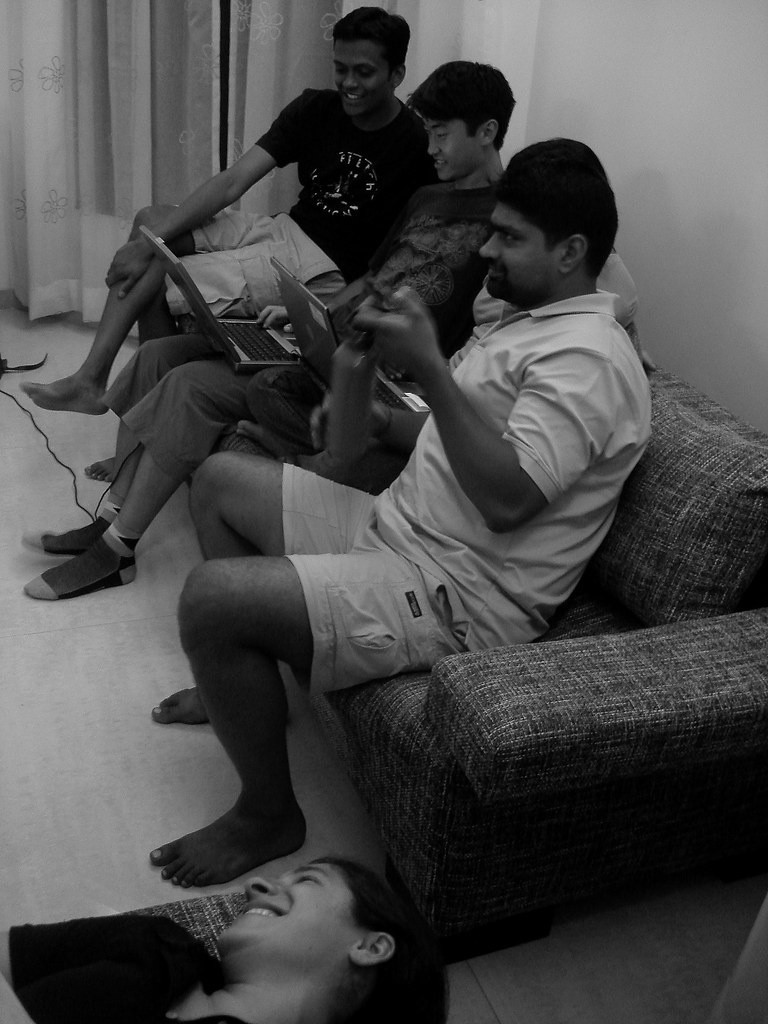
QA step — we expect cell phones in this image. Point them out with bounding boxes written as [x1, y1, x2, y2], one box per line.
[365, 278, 396, 312]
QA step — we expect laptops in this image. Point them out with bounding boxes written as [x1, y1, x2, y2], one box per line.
[269, 257, 431, 412]
[137, 226, 301, 372]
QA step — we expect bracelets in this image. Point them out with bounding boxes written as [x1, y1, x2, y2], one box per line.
[370, 399, 394, 440]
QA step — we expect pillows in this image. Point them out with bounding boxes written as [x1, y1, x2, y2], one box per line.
[586, 367, 768, 628]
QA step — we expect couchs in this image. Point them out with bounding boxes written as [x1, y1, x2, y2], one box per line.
[0, 207, 768, 1024]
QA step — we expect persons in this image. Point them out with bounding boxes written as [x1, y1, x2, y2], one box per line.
[19, 58, 518, 597]
[18, 5, 457, 479]
[0, 854, 450, 1024]
[232, 241, 648, 498]
[148, 134, 657, 888]
